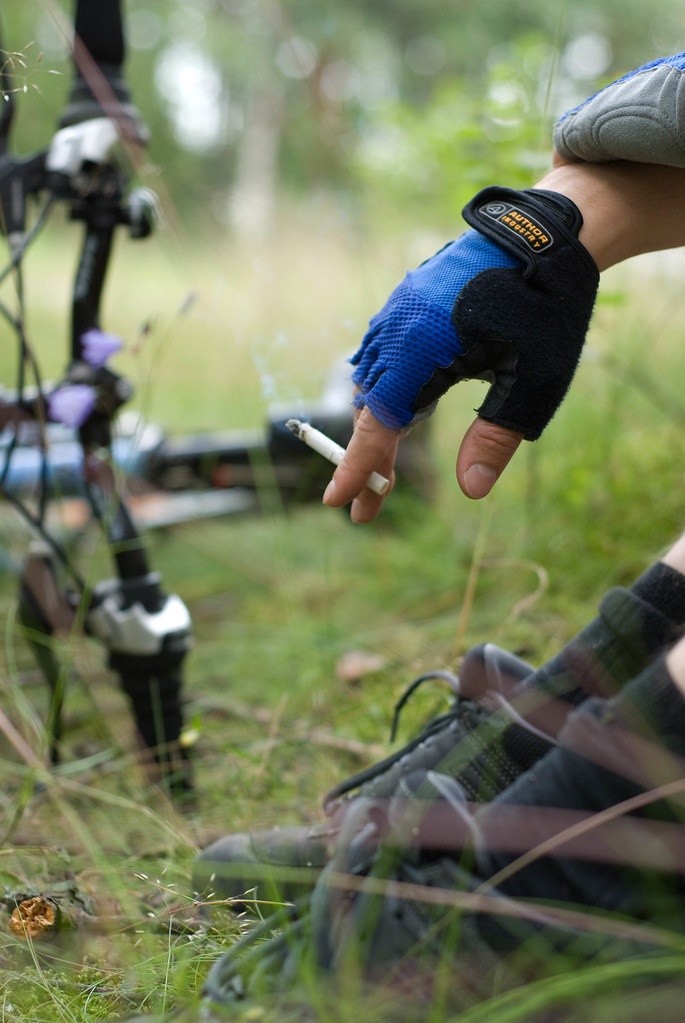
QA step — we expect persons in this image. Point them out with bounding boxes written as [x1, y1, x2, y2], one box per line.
[194, 50, 685, 1022]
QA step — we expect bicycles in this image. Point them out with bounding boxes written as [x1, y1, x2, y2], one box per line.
[0, 114, 448, 812]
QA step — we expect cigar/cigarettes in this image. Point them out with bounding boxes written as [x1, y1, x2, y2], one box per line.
[286, 418, 389, 495]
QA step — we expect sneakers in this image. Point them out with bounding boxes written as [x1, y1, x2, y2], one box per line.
[206, 770, 685, 1022]
[195, 642, 569, 917]
